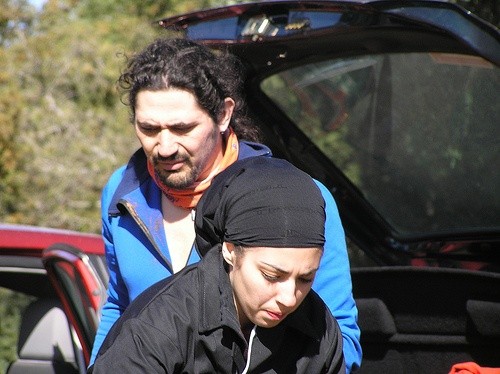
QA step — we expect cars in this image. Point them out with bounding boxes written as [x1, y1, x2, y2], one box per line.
[0, 221, 121, 374]
[153, 0, 499, 374]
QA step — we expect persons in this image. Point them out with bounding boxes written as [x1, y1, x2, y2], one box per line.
[86, 156, 346, 374]
[86, 38, 364, 374]
